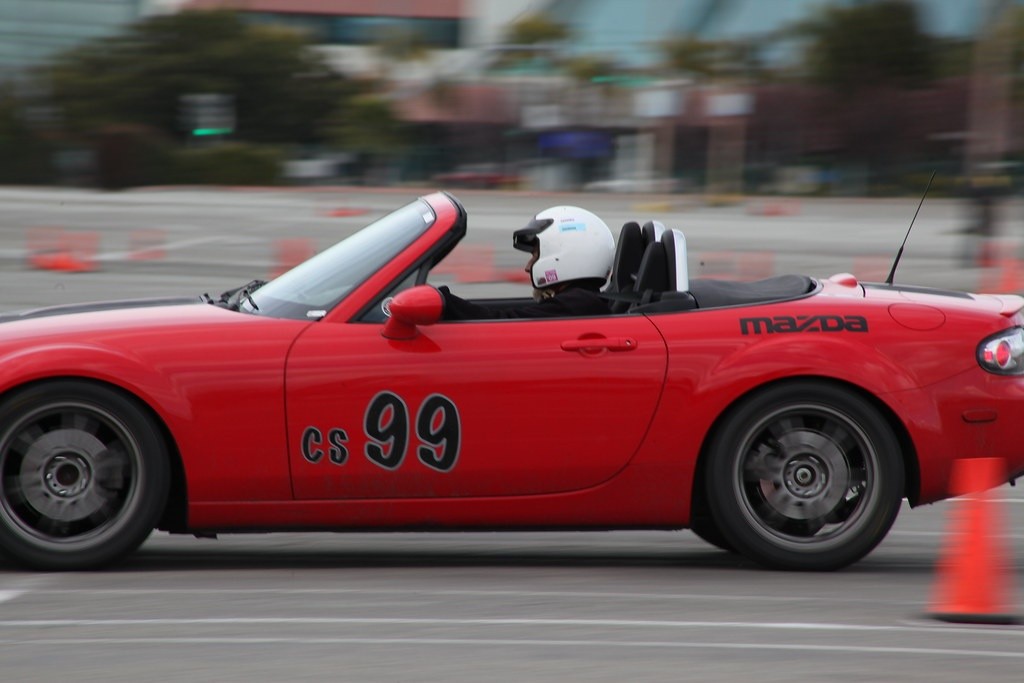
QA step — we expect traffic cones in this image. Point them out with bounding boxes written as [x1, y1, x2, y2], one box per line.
[926, 459, 1024, 623]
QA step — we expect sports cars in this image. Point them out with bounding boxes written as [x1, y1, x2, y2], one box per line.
[0, 186, 1024, 573]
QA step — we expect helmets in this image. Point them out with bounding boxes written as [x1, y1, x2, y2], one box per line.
[512, 206, 615, 289]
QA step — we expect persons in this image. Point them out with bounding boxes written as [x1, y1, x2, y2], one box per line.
[438, 205, 615, 319]
